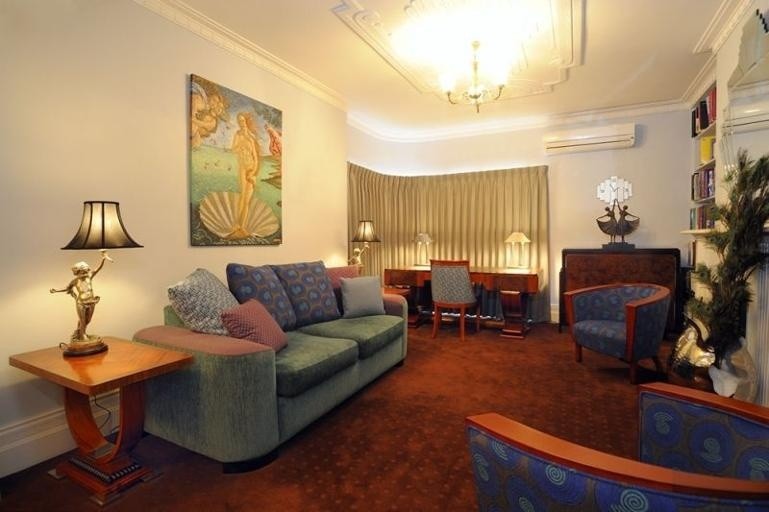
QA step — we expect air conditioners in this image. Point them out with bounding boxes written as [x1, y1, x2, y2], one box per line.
[544, 123, 637, 153]
[720, 99, 769, 136]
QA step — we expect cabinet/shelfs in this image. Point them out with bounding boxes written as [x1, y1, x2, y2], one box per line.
[679, 81, 715, 303]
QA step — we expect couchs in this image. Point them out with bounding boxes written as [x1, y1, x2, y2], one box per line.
[131, 258, 409, 464]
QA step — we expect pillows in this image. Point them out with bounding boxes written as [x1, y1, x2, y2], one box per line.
[272, 259, 341, 325]
[226, 262, 297, 332]
[338, 273, 386, 318]
[168, 267, 239, 337]
[219, 298, 289, 353]
[325, 264, 359, 287]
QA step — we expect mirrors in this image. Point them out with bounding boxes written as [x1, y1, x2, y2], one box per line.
[722, 78, 769, 225]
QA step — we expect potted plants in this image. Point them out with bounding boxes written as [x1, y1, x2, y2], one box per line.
[699, 150, 769, 403]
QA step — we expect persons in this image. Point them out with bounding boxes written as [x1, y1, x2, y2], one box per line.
[50, 258, 105, 340]
[232, 112, 258, 229]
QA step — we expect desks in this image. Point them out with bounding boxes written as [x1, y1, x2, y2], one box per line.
[384, 287, 411, 296]
[9, 336, 195, 504]
[384, 263, 544, 341]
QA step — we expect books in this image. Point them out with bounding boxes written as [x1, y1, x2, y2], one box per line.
[685, 86, 716, 291]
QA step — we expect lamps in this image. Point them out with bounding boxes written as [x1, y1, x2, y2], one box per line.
[50, 200, 144, 357]
[503, 232, 532, 269]
[348, 220, 383, 265]
[412, 232, 434, 266]
[441, 38, 507, 114]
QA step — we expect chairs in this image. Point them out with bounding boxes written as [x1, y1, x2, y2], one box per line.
[563, 282, 673, 383]
[466, 382, 768, 510]
[429, 258, 481, 341]
